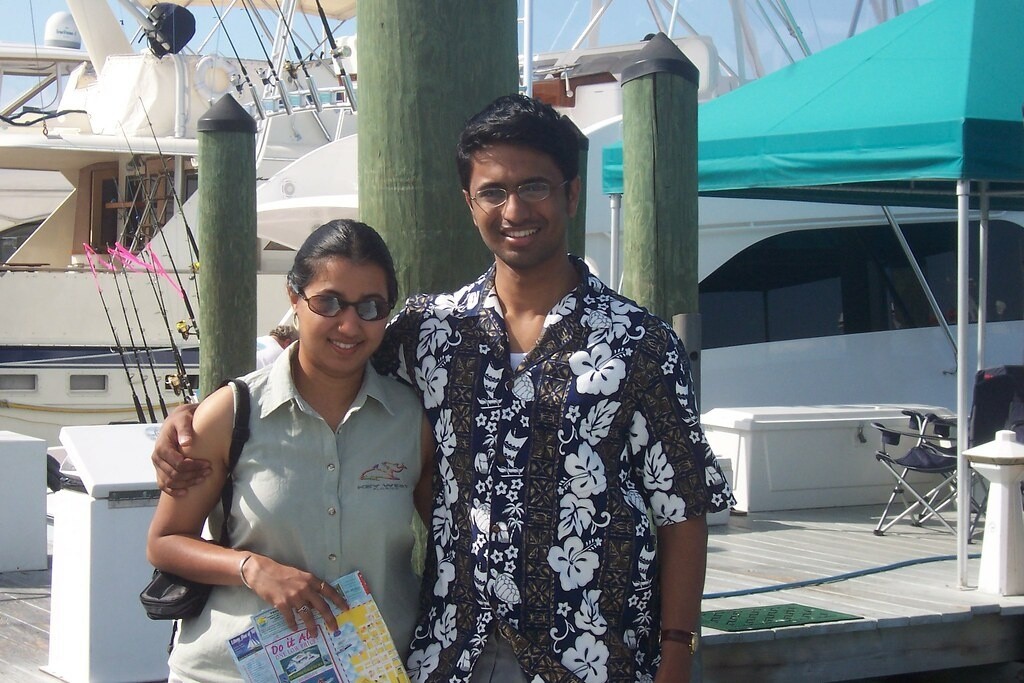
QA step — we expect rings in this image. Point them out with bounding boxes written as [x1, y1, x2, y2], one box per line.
[320, 581, 325, 590]
[298, 606, 307, 612]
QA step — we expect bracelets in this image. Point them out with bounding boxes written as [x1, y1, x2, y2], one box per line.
[239, 555, 252, 589]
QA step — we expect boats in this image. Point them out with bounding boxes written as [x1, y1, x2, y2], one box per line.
[0, 0, 1023, 447]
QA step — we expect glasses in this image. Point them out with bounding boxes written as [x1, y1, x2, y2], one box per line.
[467, 179, 569, 208]
[289, 280, 391, 321]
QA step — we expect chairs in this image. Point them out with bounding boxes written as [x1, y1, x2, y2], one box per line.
[872, 365, 1024, 546]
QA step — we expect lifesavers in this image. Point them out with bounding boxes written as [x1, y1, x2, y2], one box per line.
[194, 53, 243, 105]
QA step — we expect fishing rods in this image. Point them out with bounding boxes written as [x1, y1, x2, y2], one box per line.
[209, 0, 359, 120]
[81, 95, 199, 424]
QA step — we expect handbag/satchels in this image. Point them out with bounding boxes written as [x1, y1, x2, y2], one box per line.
[140, 378, 251, 620]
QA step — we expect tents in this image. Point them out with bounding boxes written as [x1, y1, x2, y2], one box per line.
[602, 0, 1024, 589]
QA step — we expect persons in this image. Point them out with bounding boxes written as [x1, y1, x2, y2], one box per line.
[153, 94, 736, 683]
[146, 217, 440, 683]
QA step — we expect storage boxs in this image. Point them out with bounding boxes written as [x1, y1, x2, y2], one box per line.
[698, 403, 961, 513]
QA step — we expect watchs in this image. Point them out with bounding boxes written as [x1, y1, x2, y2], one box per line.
[660, 629, 700, 654]
